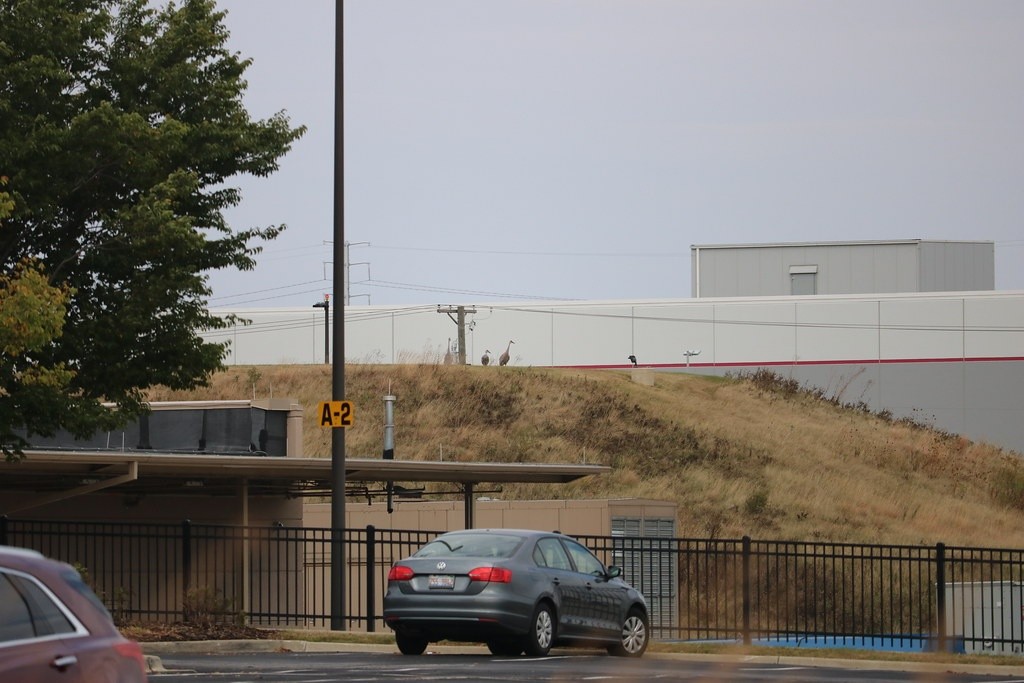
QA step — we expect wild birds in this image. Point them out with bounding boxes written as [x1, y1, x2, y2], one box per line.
[443, 337, 453, 366]
[498, 340, 516, 367]
[480, 350, 492, 365]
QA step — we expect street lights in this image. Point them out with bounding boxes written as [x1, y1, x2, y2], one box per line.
[311, 298, 332, 365]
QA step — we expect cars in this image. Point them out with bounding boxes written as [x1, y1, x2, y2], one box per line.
[1, 545, 150, 683]
[380, 527, 651, 659]
